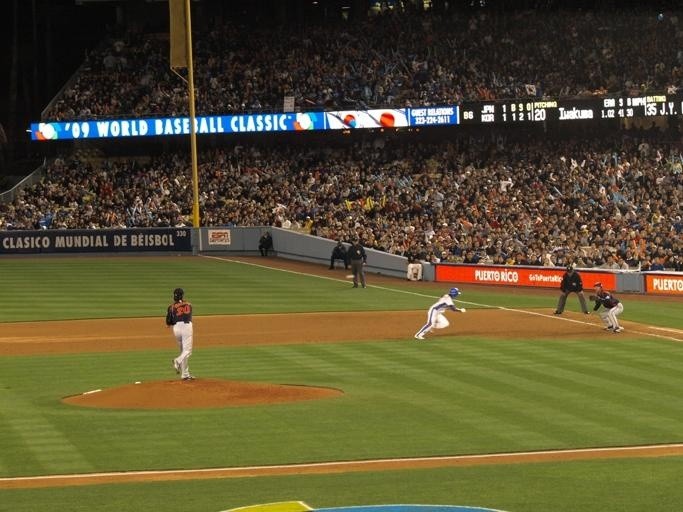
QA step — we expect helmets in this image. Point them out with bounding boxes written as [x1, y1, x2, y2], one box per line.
[449, 288, 461, 295]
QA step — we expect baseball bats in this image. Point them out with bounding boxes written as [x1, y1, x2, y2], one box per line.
[62, 389, 102, 398]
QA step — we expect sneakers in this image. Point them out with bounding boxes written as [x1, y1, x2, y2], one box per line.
[182, 375, 197, 379]
[415, 336, 424, 339]
[172, 359, 180, 374]
[606, 326, 624, 333]
[429, 331, 433, 333]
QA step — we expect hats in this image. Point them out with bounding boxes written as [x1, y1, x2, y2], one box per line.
[174, 288, 183, 299]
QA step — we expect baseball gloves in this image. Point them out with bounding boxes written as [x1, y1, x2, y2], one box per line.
[590, 295, 600, 301]
[560, 280, 568, 292]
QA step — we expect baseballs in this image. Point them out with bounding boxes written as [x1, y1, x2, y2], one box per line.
[461, 308, 466, 312]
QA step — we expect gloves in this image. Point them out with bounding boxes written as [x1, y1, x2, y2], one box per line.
[461, 308, 465, 312]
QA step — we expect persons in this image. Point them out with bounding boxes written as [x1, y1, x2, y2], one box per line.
[554, 265, 590, 316]
[589, 282, 626, 332]
[415, 288, 468, 341]
[330, 238, 369, 288]
[165, 289, 195, 382]
